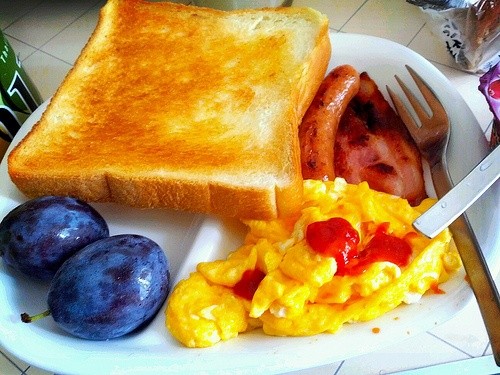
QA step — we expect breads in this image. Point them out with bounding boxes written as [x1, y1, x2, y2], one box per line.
[8, 0, 329, 220]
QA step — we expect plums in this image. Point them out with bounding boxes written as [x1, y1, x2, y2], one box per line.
[1, 194, 169, 340]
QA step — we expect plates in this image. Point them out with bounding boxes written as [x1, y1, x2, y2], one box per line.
[0, 31, 500, 374]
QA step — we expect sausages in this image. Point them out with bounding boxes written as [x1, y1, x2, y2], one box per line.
[298, 63, 361, 184]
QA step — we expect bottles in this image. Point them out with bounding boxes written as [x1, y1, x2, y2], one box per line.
[0, 29, 44, 143]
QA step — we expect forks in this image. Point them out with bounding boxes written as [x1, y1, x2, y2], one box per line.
[385, 63, 500, 369]
